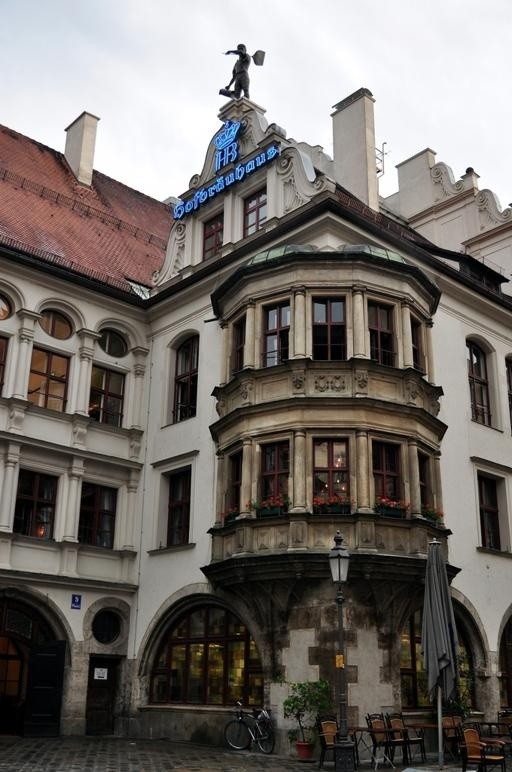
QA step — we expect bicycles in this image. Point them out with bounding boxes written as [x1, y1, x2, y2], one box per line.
[223, 698, 279, 755]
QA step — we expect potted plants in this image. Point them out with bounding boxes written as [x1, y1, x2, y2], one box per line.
[280, 676, 338, 761]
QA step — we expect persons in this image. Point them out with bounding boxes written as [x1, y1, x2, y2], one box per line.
[224, 44, 251, 99]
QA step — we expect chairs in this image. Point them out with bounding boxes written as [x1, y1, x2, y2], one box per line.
[314, 707, 512, 772]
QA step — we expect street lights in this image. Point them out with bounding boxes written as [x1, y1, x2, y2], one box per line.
[327, 529, 357, 769]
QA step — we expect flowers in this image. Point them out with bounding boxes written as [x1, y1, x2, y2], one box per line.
[220, 493, 446, 527]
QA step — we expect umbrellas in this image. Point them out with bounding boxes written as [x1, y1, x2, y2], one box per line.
[421, 537, 462, 771]
